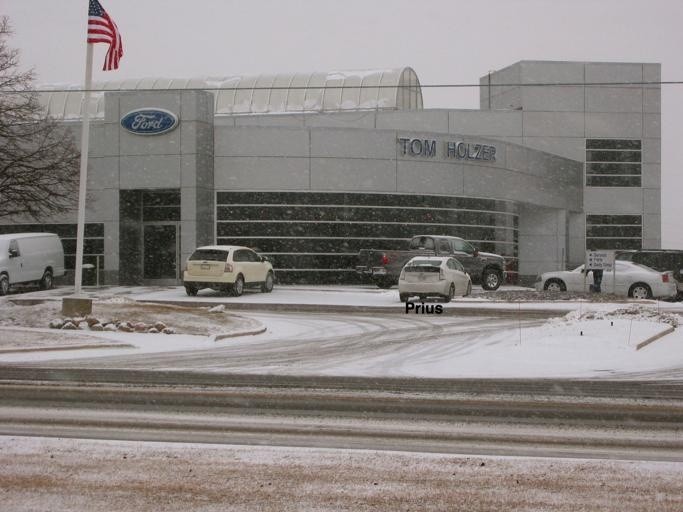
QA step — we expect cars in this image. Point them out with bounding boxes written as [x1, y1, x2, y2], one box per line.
[532, 257, 677, 300]
[397, 256, 472, 303]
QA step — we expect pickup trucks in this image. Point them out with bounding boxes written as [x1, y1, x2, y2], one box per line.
[353, 233, 507, 291]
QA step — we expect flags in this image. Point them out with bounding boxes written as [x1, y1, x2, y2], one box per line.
[84, 1, 123, 73]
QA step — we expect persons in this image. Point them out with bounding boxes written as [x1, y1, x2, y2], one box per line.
[580, 246, 603, 287]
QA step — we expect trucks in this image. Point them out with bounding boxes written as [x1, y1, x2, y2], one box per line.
[0, 230, 69, 297]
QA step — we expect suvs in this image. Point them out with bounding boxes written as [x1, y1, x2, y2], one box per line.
[611, 248, 682, 304]
[182, 245, 276, 298]
